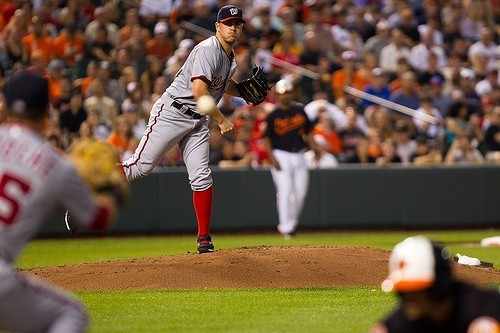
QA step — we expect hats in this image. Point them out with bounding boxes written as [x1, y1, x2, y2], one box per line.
[217, 5, 246, 24]
[381, 234, 454, 292]
[2, 71, 49, 114]
[275, 80, 291, 94]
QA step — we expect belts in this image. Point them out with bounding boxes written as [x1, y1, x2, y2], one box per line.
[171, 101, 203, 119]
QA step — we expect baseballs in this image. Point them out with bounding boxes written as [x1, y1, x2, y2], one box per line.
[198, 97, 218, 113]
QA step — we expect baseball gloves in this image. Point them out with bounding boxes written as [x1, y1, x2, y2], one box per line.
[70, 139, 131, 209]
[234, 65, 271, 105]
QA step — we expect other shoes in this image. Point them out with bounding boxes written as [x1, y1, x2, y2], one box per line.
[197, 233, 214, 253]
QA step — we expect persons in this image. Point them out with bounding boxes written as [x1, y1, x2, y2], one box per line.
[259, 78, 319, 242]
[367, 235, 500, 333]
[0, 0, 500, 168]
[0, 69, 122, 333]
[115, 4, 254, 253]
